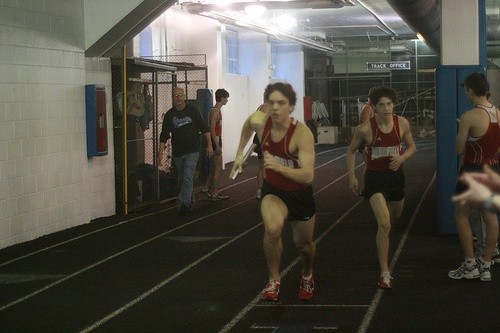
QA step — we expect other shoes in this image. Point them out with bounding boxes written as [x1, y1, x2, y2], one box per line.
[257, 188, 262, 198]
[180, 202, 195, 215]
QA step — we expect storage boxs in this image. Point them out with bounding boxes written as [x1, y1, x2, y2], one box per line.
[317, 126, 338, 145]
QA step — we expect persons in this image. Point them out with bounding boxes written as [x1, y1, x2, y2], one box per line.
[202, 89, 229, 200]
[230, 83, 315, 299]
[447, 74, 500, 280]
[158, 88, 214, 216]
[346, 87, 417, 288]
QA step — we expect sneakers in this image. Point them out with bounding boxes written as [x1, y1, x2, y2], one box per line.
[298, 276, 315, 300]
[447, 258, 493, 281]
[380, 275, 392, 288]
[260, 279, 280, 299]
[202, 186, 211, 193]
[208, 193, 229, 202]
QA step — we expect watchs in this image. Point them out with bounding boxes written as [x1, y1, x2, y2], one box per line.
[484, 194, 494, 207]
